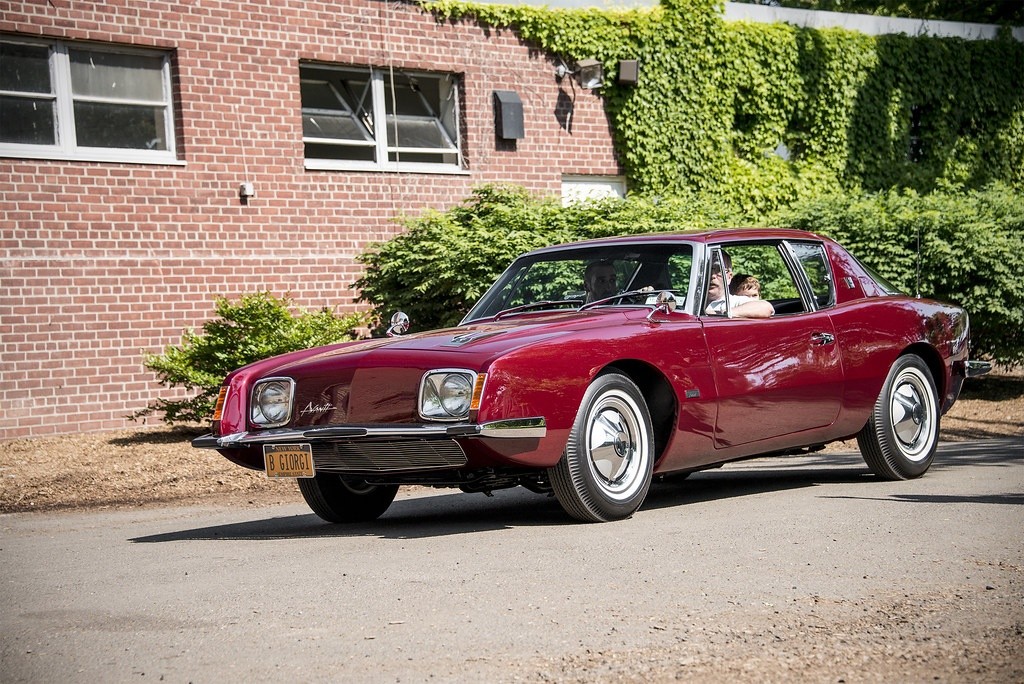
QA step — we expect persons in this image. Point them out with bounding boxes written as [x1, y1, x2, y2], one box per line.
[729, 272, 762, 300]
[635, 248, 775, 318]
[565, 262, 636, 309]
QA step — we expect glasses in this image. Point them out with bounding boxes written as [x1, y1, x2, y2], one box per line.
[712, 266, 730, 274]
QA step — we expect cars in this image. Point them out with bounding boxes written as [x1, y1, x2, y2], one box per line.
[190, 228, 993, 524]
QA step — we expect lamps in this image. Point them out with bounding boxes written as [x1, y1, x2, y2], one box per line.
[557, 58, 605, 89]
